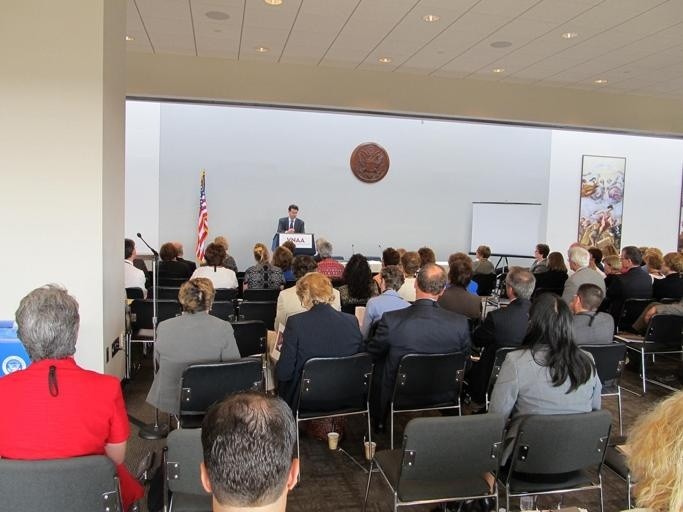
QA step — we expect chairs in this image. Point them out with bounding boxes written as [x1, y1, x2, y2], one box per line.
[169, 356, 266, 429]
[238, 277, 245, 300]
[613, 312, 677, 399]
[484, 345, 521, 411]
[362, 410, 512, 512]
[236, 270, 247, 278]
[499, 408, 615, 512]
[147, 285, 181, 300]
[127, 300, 183, 379]
[126, 286, 145, 299]
[1, 455, 141, 512]
[292, 350, 374, 484]
[389, 342, 475, 450]
[212, 287, 238, 302]
[603, 434, 635, 511]
[239, 300, 277, 332]
[330, 276, 350, 290]
[575, 342, 627, 436]
[659, 296, 677, 306]
[283, 279, 297, 291]
[243, 288, 283, 302]
[210, 300, 236, 322]
[616, 296, 652, 334]
[227, 320, 267, 360]
[161, 428, 214, 512]
[342, 303, 368, 316]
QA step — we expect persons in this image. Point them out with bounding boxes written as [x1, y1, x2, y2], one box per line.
[314, 238, 343, 262]
[590, 247, 604, 277]
[277, 205, 305, 233]
[362, 265, 409, 340]
[398, 252, 419, 300]
[188, 245, 238, 288]
[600, 255, 621, 312]
[578, 205, 621, 246]
[124, 239, 148, 298]
[559, 247, 605, 305]
[199, 391, 300, 512]
[275, 274, 372, 454]
[482, 294, 603, 499]
[613, 247, 652, 328]
[329, 254, 381, 308]
[144, 279, 241, 415]
[472, 268, 539, 404]
[317, 242, 345, 278]
[625, 393, 682, 511]
[368, 264, 470, 434]
[438, 262, 482, 320]
[282, 241, 296, 252]
[652, 253, 683, 302]
[0, 284, 145, 511]
[470, 248, 495, 277]
[173, 243, 196, 271]
[443, 254, 480, 292]
[271, 247, 295, 281]
[416, 248, 434, 264]
[374, 250, 403, 290]
[213, 237, 237, 274]
[273, 255, 341, 334]
[641, 249, 665, 287]
[568, 283, 614, 346]
[532, 253, 567, 294]
[243, 243, 284, 292]
[644, 300, 683, 324]
[526, 245, 547, 276]
[145, 243, 193, 291]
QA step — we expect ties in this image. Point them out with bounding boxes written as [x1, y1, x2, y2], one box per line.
[290, 218, 293, 228]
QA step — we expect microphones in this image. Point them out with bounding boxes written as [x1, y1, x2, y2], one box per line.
[135, 233, 159, 258]
[378, 244, 383, 252]
[352, 244, 355, 255]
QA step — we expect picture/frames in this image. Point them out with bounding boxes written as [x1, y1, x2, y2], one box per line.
[577, 154, 626, 264]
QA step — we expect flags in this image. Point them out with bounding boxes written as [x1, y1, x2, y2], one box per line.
[194, 171, 209, 263]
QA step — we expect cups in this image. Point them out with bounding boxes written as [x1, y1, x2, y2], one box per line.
[364, 441, 377, 460]
[327, 432, 340, 451]
